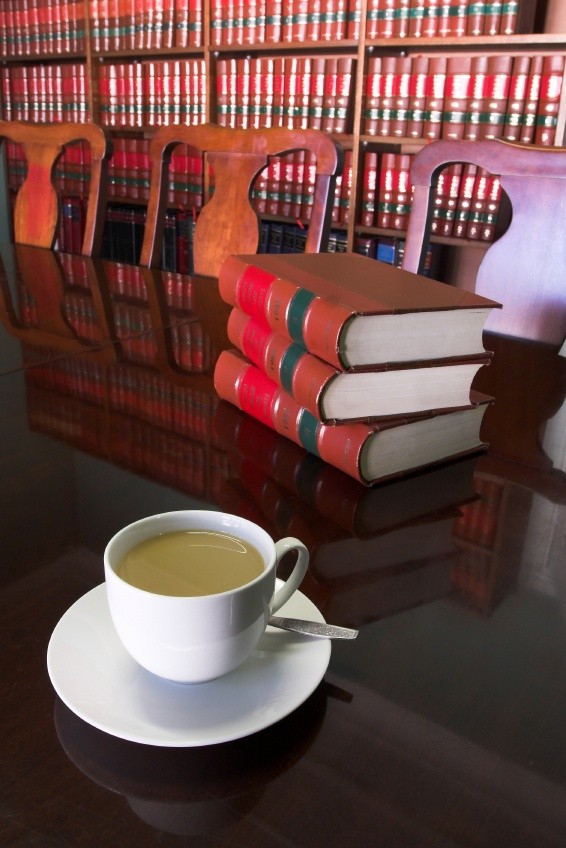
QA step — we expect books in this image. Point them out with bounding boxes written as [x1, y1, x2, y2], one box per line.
[215, 253, 499, 486]
[0, 0, 566, 296]
[210, 397, 488, 628]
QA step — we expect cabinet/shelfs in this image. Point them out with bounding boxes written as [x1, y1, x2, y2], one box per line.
[0, 0, 566, 250]
[12, 244, 534, 622]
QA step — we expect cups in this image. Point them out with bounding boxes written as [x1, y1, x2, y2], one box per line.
[103, 509, 310, 685]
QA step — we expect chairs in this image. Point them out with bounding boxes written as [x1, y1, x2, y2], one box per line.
[399, 132, 565, 345]
[0, 245, 121, 367]
[0, 121, 112, 255]
[140, 125, 341, 278]
[471, 331, 566, 506]
[140, 267, 233, 388]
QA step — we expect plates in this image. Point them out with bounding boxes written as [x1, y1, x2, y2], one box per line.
[46, 576, 332, 748]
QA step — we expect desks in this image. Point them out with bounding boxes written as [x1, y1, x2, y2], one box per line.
[1, 241, 566, 848]
